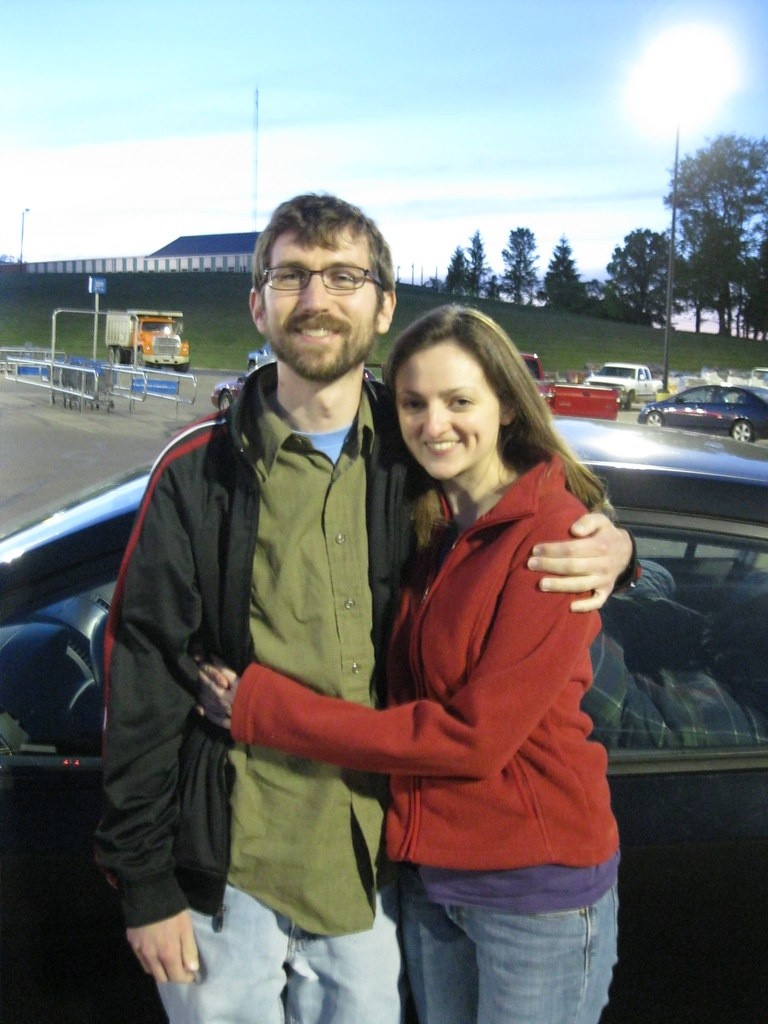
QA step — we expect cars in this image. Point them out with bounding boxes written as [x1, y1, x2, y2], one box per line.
[1, 415, 768, 1023]
[637, 385, 768, 444]
[210, 370, 251, 412]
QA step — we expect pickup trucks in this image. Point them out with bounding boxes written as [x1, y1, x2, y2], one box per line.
[519, 352, 621, 421]
[584, 361, 664, 413]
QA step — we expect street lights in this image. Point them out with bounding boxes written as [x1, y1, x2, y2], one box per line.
[20, 209, 31, 265]
[636, 43, 731, 394]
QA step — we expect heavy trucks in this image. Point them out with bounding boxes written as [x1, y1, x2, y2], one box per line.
[105, 309, 191, 373]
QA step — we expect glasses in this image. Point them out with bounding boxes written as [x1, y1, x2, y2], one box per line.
[260, 264, 388, 291]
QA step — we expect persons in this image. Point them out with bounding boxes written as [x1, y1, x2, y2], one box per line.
[94, 194, 643, 1024]
[194, 305, 619, 1023]
